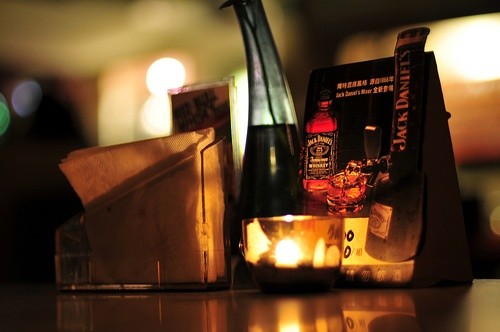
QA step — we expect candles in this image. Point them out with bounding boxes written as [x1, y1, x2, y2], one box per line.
[241, 214, 345, 292]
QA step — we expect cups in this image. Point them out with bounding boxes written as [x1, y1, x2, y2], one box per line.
[325, 170, 368, 209]
[241, 215, 347, 292]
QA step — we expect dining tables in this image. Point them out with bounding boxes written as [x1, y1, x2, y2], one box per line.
[0, 277, 500, 331]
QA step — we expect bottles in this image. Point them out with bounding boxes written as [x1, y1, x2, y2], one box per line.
[302, 89, 339, 193]
